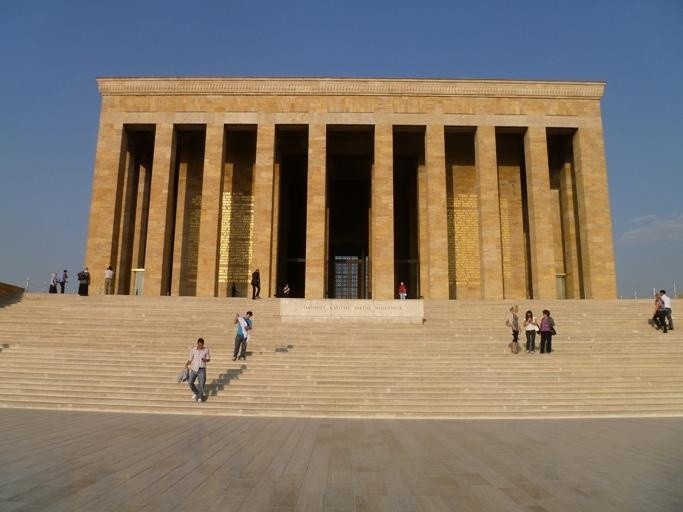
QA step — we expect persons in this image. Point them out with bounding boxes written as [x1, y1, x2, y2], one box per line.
[232, 311, 252, 361]
[185, 338, 210, 403]
[60, 270, 68, 293]
[399, 282, 406, 300]
[78, 267, 90, 296]
[653, 294, 667, 333]
[510, 306, 555, 354]
[251, 269, 262, 299]
[660, 290, 673, 330]
[49, 273, 58, 293]
[104, 266, 114, 295]
[284, 284, 290, 297]
[232, 282, 237, 297]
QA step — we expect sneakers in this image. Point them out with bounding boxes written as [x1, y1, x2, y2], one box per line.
[197, 399, 202, 403]
[231, 357, 236, 361]
[240, 356, 244, 360]
[191, 394, 196, 399]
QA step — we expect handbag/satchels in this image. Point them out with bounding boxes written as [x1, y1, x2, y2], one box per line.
[505, 320, 511, 327]
[550, 328, 555, 335]
